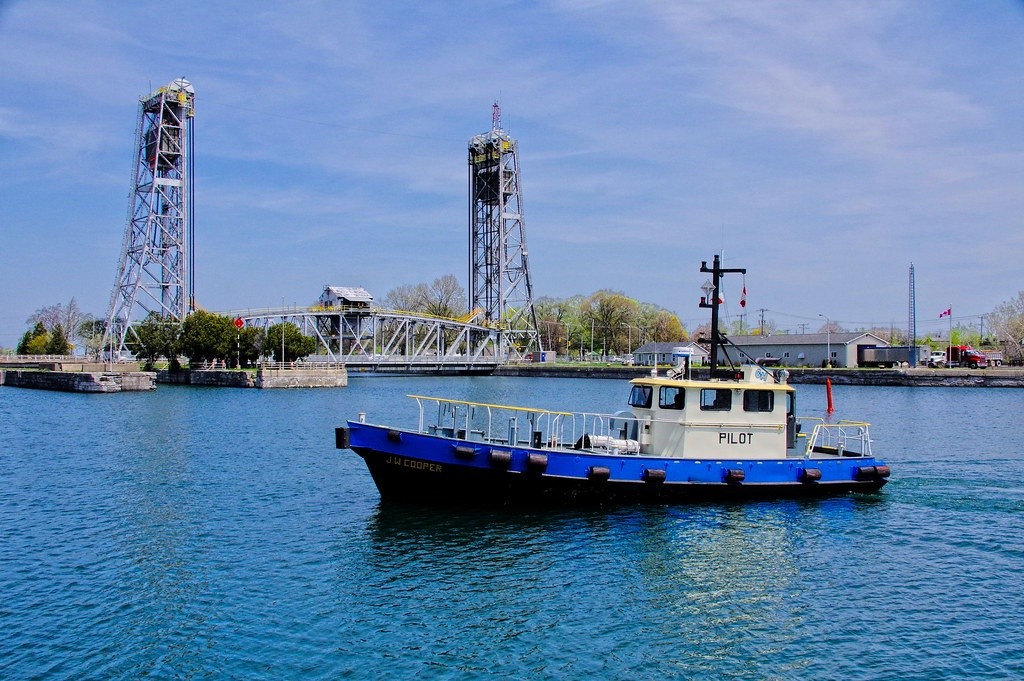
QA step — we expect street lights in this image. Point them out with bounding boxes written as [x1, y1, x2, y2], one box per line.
[818, 313, 831, 368]
[622, 323, 632, 367]
[588, 318, 594, 364]
[560, 320, 569, 363]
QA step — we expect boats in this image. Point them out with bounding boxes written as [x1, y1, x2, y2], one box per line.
[335, 249, 890, 500]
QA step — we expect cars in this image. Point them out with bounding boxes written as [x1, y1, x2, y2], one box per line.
[921, 351, 946, 366]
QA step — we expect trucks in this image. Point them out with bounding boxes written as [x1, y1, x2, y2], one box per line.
[945, 345, 989, 369]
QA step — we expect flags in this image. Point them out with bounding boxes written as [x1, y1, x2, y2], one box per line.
[938, 308, 949, 321]
